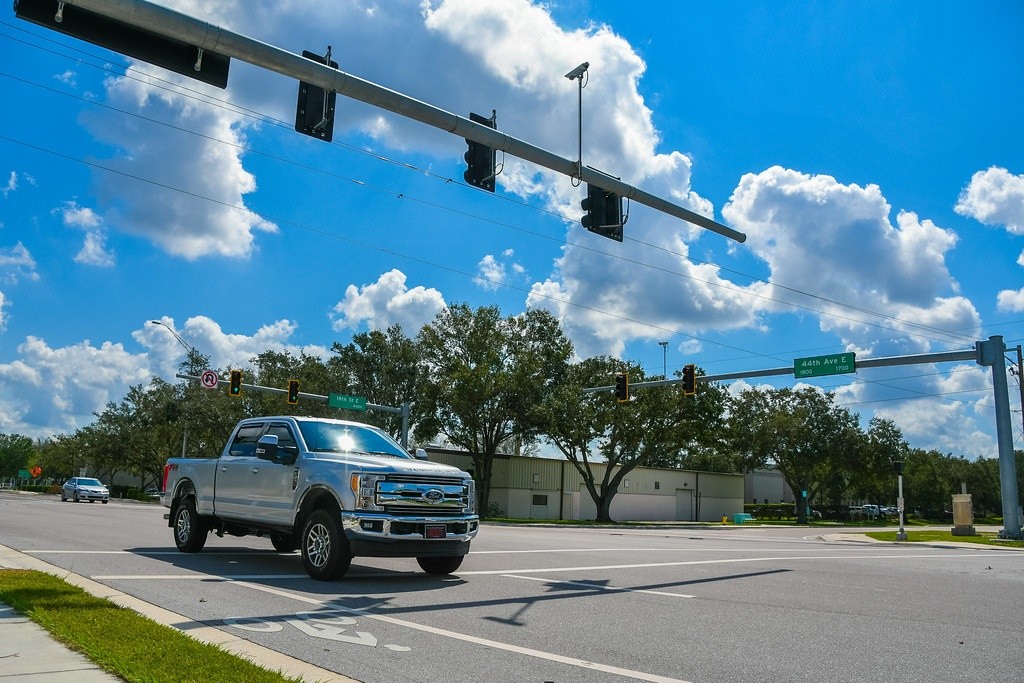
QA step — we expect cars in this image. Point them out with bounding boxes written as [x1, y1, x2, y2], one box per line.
[60, 477, 110, 502]
[863, 503, 904, 518]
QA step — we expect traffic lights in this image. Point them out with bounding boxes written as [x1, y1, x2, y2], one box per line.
[681, 364, 695, 395]
[894, 461, 903, 474]
[615, 373, 628, 401]
[230, 369, 242, 396]
[288, 380, 299, 404]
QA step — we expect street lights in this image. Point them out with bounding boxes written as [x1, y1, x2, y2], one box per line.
[152, 320, 196, 457]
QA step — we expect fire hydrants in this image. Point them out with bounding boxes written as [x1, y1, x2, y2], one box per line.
[721, 513, 729, 525]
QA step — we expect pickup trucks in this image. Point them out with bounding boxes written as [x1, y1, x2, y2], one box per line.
[162, 414, 476, 581]
